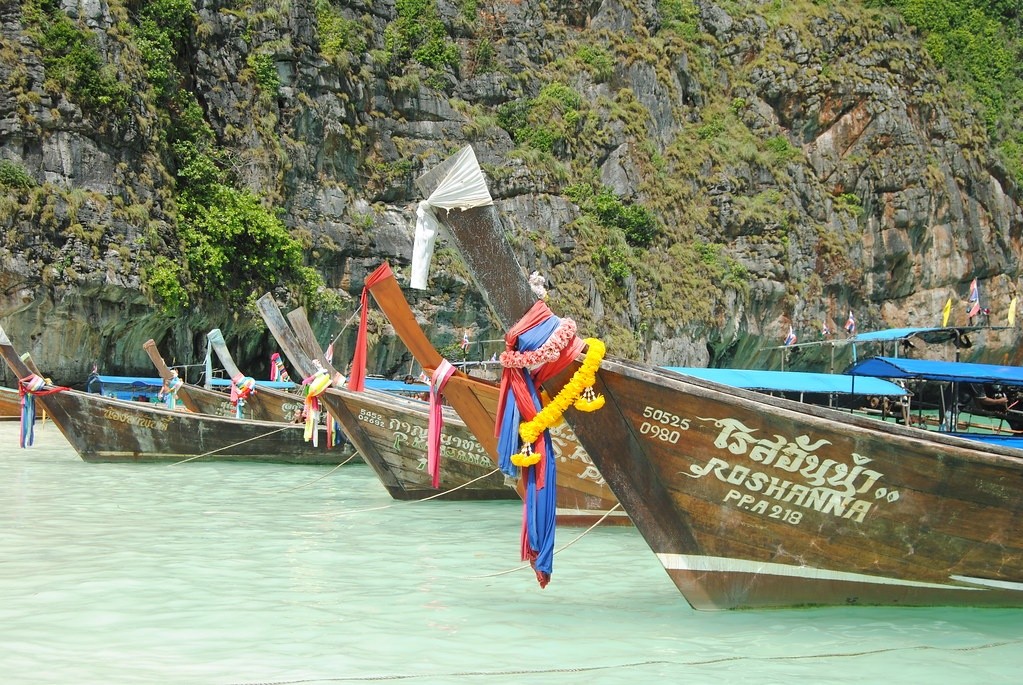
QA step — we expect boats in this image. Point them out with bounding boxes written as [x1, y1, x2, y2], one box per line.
[0, 260, 1023, 527]
[415, 144, 1023, 613]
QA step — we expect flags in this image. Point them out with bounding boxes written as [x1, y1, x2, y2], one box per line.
[942, 300, 951, 327]
[822, 323, 830, 335]
[844, 311, 855, 333]
[968, 280, 978, 318]
[786, 329, 792, 344]
[1008, 299, 1016, 325]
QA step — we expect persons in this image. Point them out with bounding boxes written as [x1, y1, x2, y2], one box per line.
[899, 387, 910, 425]
[945, 383, 1008, 412]
[815, 329, 823, 341]
[846, 323, 852, 336]
[784, 337, 787, 345]
